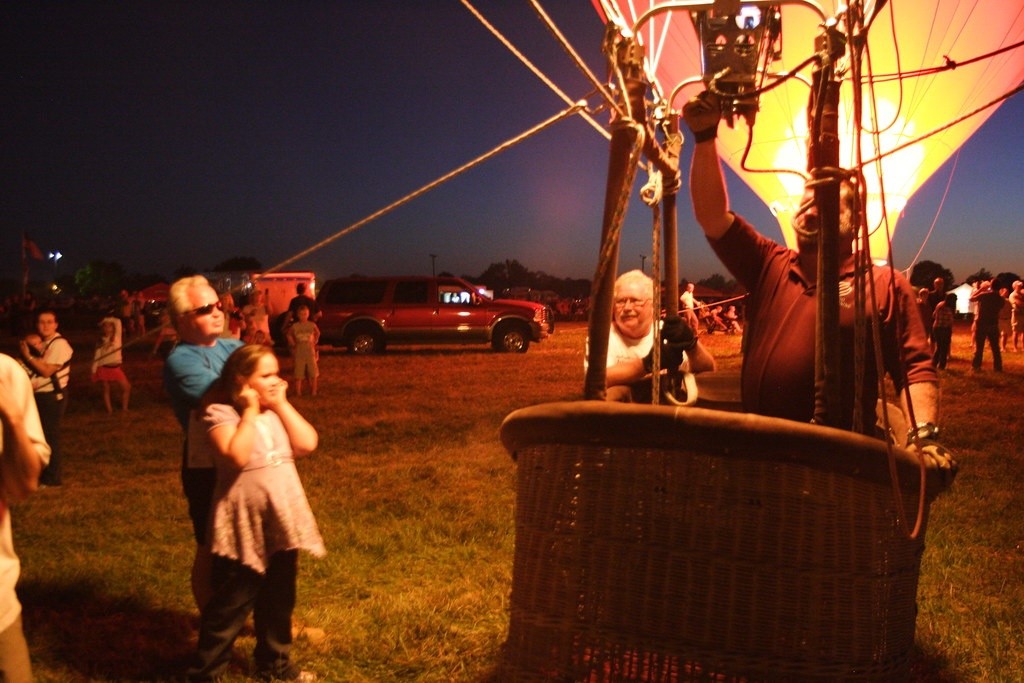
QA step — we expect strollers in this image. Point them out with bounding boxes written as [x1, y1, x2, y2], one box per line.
[699, 300, 743, 336]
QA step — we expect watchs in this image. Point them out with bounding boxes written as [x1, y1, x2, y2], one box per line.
[907, 421, 939, 442]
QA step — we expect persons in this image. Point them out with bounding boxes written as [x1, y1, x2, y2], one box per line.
[0, 351, 51, 683]
[679, 282, 743, 336]
[0, 282, 321, 491]
[583, 270, 715, 405]
[910, 276, 1024, 372]
[185, 343, 327, 681]
[548, 296, 590, 321]
[682, 90, 960, 492]
[163, 274, 256, 636]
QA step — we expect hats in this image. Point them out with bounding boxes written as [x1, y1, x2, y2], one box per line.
[1012, 281, 1023, 290]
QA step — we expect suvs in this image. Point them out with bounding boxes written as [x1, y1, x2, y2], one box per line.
[310, 271, 554, 356]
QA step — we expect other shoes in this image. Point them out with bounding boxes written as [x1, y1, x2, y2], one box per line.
[39, 472, 64, 487]
[270, 671, 316, 683]
[1000, 347, 1007, 353]
[969, 367, 981, 373]
[291, 623, 326, 643]
[1013, 347, 1018, 352]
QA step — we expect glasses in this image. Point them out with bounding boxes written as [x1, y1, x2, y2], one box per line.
[182, 301, 224, 315]
[613, 295, 654, 306]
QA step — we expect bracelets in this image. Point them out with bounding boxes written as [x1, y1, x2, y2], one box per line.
[693, 125, 717, 143]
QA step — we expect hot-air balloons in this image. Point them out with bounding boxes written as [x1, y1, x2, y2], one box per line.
[499, 0, 1024, 683]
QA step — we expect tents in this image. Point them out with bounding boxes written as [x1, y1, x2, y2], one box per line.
[946, 282, 973, 312]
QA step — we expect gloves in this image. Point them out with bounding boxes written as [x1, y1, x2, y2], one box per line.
[662, 313, 697, 352]
[642, 337, 683, 374]
